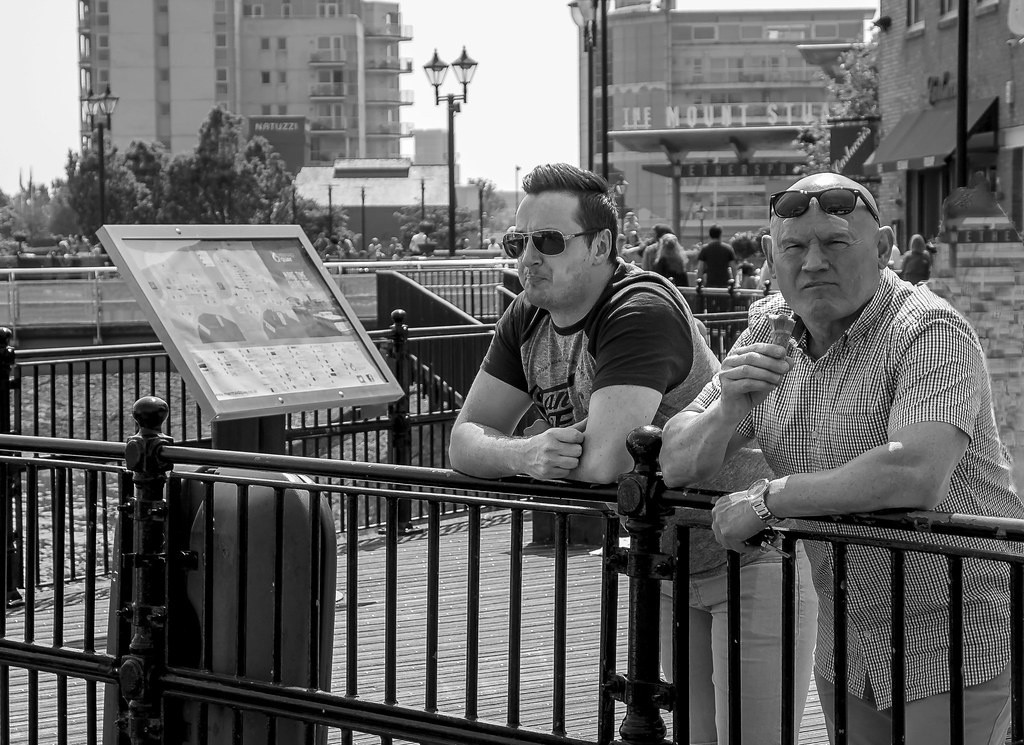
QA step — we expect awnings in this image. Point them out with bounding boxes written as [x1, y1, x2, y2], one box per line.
[862, 97, 998, 174]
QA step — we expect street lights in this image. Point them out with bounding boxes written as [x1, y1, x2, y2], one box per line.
[354, 186, 372, 251]
[81, 84, 119, 254]
[417, 177, 429, 221]
[321, 184, 340, 236]
[695, 205, 708, 245]
[616, 175, 629, 234]
[422, 46, 479, 255]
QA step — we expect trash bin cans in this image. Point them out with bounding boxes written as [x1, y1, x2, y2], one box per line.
[102, 464, 338, 745]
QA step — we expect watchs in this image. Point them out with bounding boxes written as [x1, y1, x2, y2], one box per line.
[747, 479, 781, 526]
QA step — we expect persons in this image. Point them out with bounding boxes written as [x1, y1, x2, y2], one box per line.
[696, 224, 739, 357]
[615, 223, 688, 286]
[447, 164, 818, 745]
[657, 174, 1024, 745]
[312, 232, 404, 260]
[888, 234, 931, 285]
[51, 233, 108, 256]
[488, 236, 501, 250]
[734, 234, 780, 291]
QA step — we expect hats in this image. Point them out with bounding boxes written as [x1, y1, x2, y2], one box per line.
[625, 211, 635, 218]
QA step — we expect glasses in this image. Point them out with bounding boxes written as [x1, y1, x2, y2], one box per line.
[768, 187, 881, 228]
[502, 229, 602, 259]
